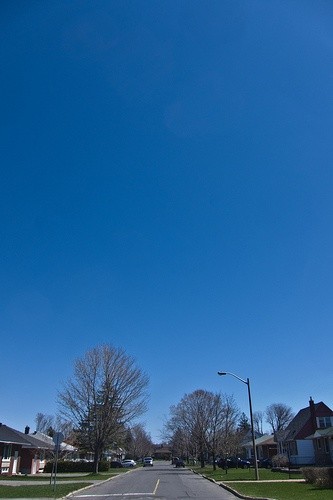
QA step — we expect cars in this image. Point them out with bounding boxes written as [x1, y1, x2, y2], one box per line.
[172, 457, 186, 468]
[143, 456, 154, 467]
[111, 459, 137, 467]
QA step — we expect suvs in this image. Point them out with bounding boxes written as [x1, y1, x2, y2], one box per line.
[217, 456, 250, 469]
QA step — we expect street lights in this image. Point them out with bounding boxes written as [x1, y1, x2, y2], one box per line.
[217, 371, 259, 480]
